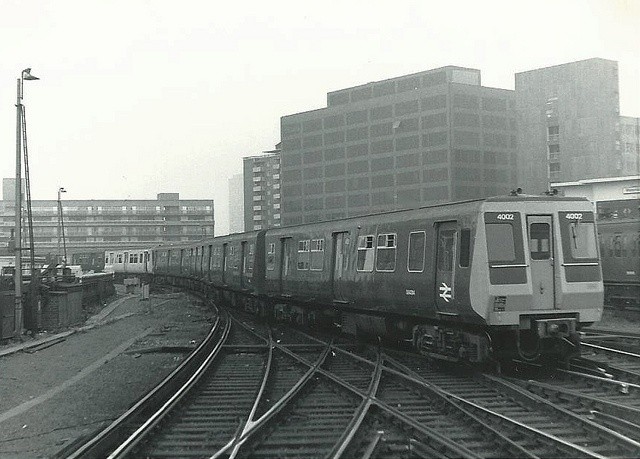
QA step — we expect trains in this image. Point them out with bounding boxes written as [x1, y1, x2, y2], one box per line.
[0, 186, 605, 371]
